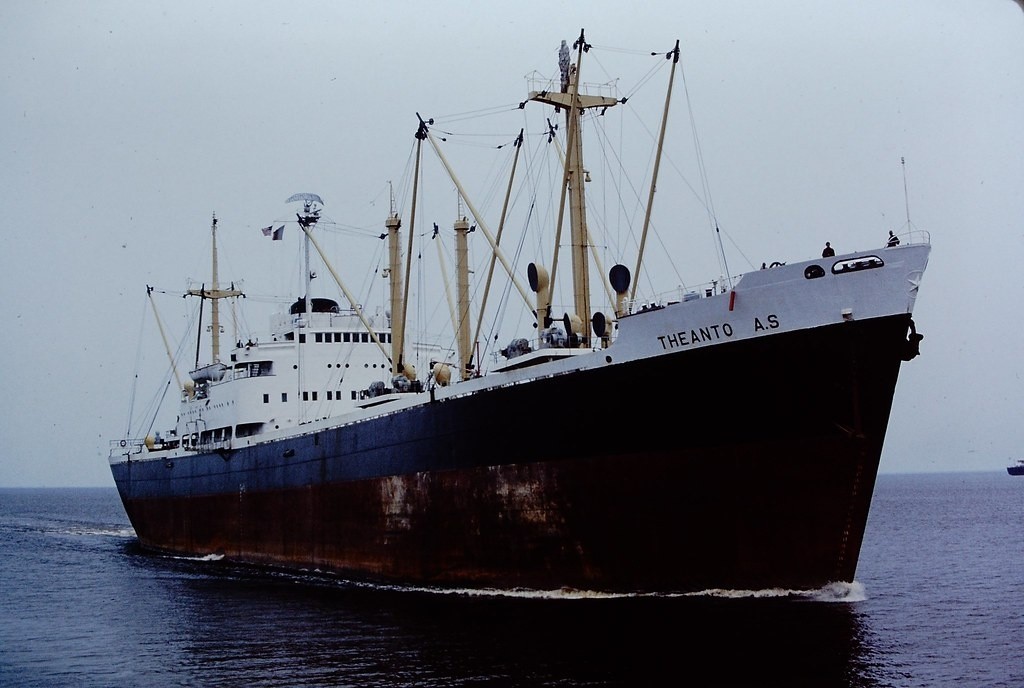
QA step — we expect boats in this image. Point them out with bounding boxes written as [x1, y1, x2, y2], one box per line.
[1006, 465, 1023, 476]
[106, 26, 932, 619]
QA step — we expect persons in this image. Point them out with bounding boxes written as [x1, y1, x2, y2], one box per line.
[821, 242, 835, 257]
[887, 230, 900, 247]
[237, 339, 253, 348]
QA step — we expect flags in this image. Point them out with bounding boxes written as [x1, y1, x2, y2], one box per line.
[261, 226, 273, 235]
[272, 225, 285, 240]
